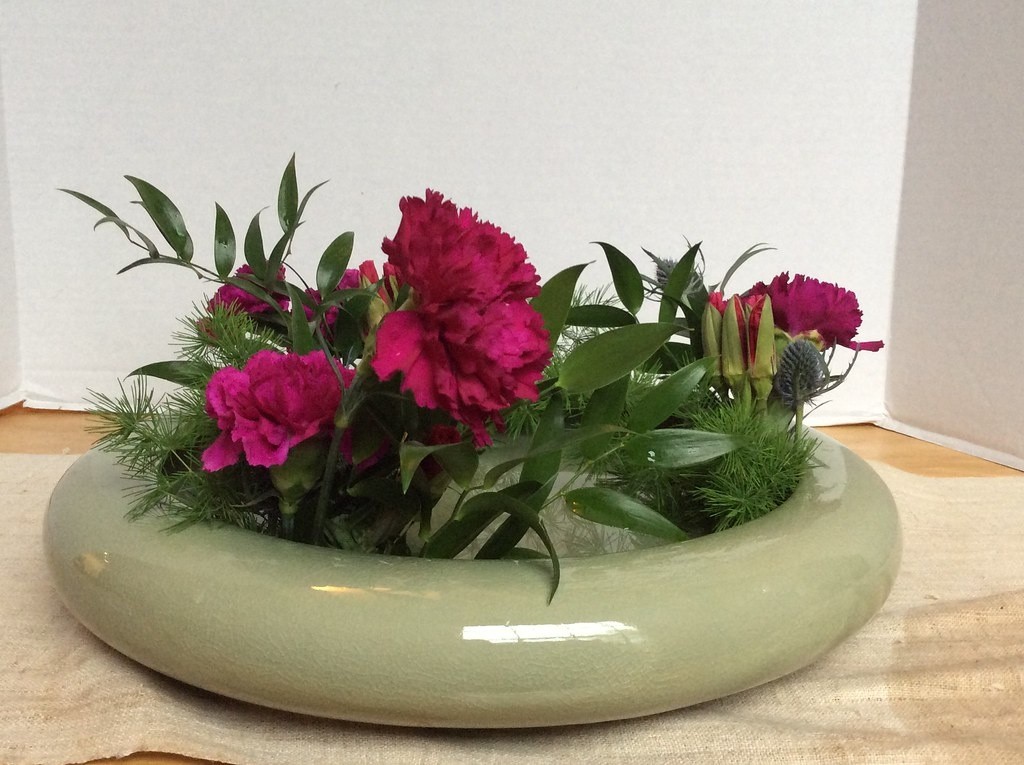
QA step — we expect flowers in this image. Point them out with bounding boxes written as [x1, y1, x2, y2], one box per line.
[195, 187, 885, 549]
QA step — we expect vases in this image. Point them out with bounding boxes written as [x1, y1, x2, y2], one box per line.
[40, 417, 903, 734]
